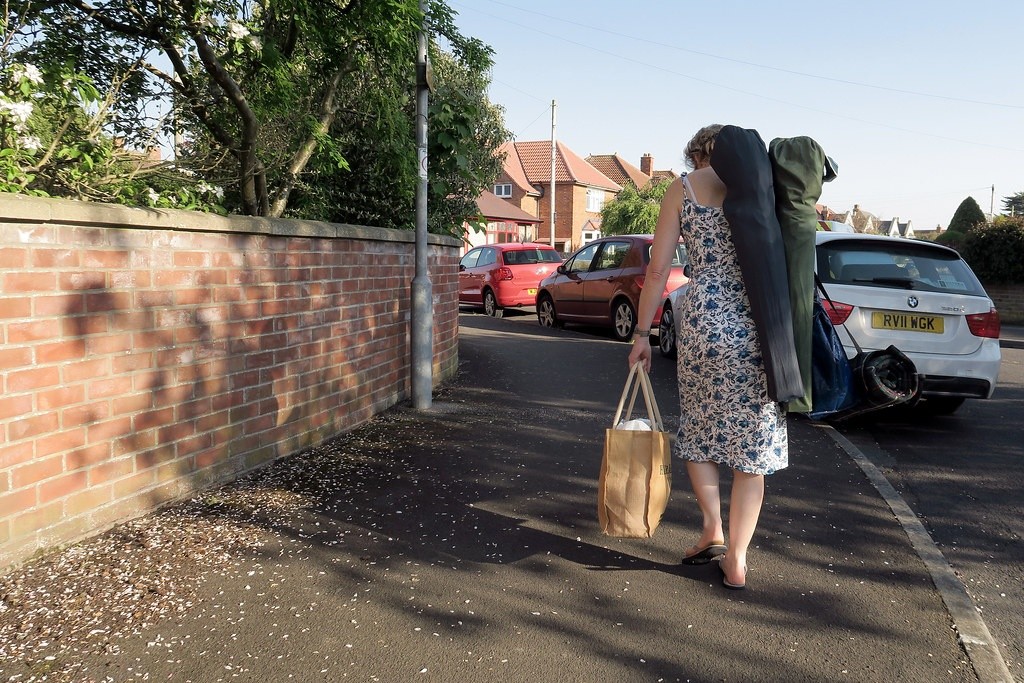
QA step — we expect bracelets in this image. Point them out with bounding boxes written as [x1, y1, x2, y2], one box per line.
[634, 325, 650, 337]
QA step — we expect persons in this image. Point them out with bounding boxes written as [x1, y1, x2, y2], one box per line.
[629, 122, 794, 589]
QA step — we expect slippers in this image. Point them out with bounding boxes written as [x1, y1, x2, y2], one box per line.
[681, 542, 727, 566]
[717, 551, 748, 591]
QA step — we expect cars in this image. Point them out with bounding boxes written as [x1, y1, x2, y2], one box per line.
[658, 230, 1001, 420]
[534, 233, 692, 343]
[458, 243, 563, 316]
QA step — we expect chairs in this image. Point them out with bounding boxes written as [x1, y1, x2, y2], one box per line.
[607, 249, 626, 268]
[515, 252, 529, 261]
[486, 253, 494, 262]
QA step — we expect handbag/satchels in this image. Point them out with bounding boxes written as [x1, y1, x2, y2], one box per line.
[807, 299, 852, 417]
[596, 357, 670, 538]
